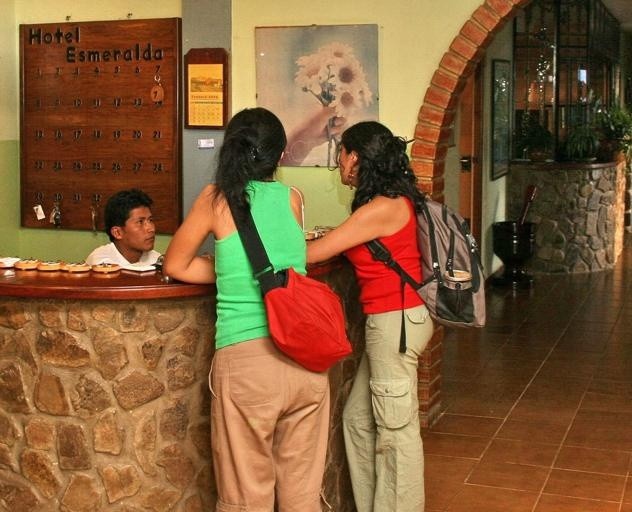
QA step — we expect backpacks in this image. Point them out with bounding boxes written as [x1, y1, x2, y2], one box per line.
[366, 188, 487, 327]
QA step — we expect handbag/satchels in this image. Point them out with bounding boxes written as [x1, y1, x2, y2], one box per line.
[259, 267, 352, 374]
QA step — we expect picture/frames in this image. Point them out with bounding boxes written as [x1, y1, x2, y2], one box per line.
[491, 57, 511, 181]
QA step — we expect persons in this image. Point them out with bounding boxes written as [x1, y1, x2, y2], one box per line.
[164, 107, 331, 512]
[306, 121, 433, 512]
[86, 190, 161, 273]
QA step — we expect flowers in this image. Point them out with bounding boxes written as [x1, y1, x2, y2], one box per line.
[294, 42, 373, 167]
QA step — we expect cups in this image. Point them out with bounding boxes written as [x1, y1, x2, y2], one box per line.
[444, 270, 472, 291]
